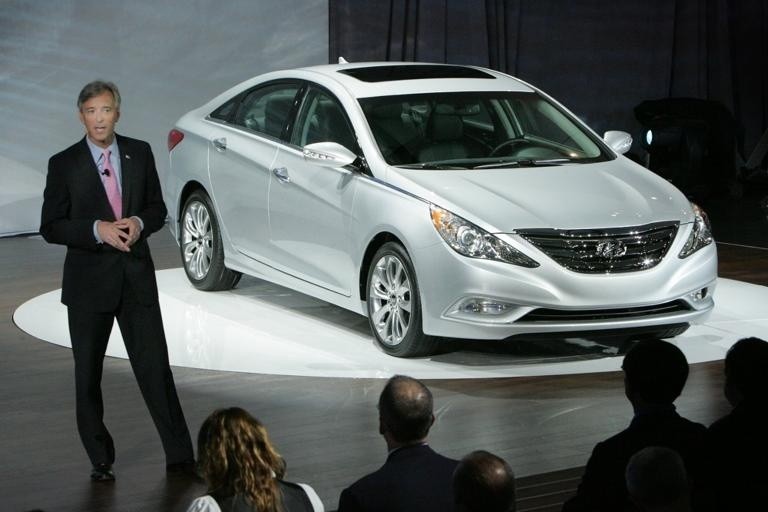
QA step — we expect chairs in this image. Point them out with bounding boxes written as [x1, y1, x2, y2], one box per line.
[266, 95, 484, 165]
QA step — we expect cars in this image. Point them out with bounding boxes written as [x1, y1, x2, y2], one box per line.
[163, 54, 719, 358]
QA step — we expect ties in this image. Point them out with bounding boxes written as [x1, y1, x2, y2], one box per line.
[101, 149, 123, 222]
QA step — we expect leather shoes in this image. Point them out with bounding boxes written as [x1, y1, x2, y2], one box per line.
[91, 460, 116, 482]
[165, 457, 206, 483]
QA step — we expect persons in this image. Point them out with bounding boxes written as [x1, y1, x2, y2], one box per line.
[562, 335, 768, 512]
[40, 79, 205, 484]
[185, 407, 325, 511]
[337, 374, 459, 511]
[452, 449, 517, 511]
